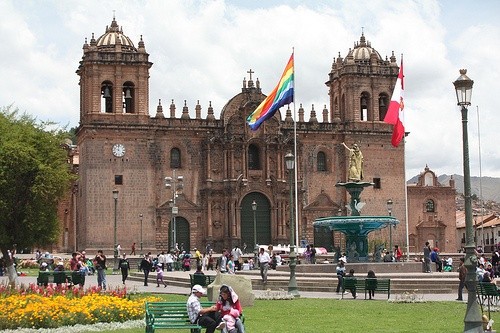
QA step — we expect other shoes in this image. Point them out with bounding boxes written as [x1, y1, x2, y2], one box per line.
[216, 322, 226, 330]
[456, 298, 463, 301]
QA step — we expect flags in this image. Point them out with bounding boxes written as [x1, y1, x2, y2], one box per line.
[384, 54, 404, 146]
[248, 46, 295, 131]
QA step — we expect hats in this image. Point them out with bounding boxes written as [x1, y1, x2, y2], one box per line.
[231, 307, 239, 318]
[433, 248, 439, 251]
[192, 285, 206, 294]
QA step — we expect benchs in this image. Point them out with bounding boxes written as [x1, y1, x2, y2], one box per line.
[145, 301, 244, 333]
[189, 274, 216, 291]
[37, 271, 85, 287]
[476, 282, 500, 306]
[342, 277, 391, 300]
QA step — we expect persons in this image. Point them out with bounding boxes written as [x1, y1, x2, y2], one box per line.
[336, 254, 349, 294]
[345, 269, 357, 299]
[186, 284, 222, 333]
[374, 244, 402, 263]
[36, 249, 94, 288]
[340, 142, 363, 181]
[258, 248, 270, 283]
[117, 243, 123, 258]
[0, 257, 17, 277]
[414, 241, 452, 274]
[305, 244, 329, 264]
[139, 242, 254, 288]
[215, 285, 245, 333]
[366, 271, 378, 300]
[117, 253, 130, 285]
[131, 243, 137, 256]
[94, 250, 108, 292]
[472, 243, 500, 307]
[456, 258, 466, 301]
[268, 244, 282, 270]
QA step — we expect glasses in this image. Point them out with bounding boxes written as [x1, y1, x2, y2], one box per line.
[220, 292, 229, 295]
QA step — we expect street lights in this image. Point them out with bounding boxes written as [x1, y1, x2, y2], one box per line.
[284, 149, 300, 298]
[452, 68, 484, 333]
[387, 199, 393, 261]
[252, 200, 258, 269]
[165, 170, 184, 255]
[139, 213, 143, 257]
[112, 187, 121, 270]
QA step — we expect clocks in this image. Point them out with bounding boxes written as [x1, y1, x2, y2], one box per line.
[112, 143, 126, 157]
